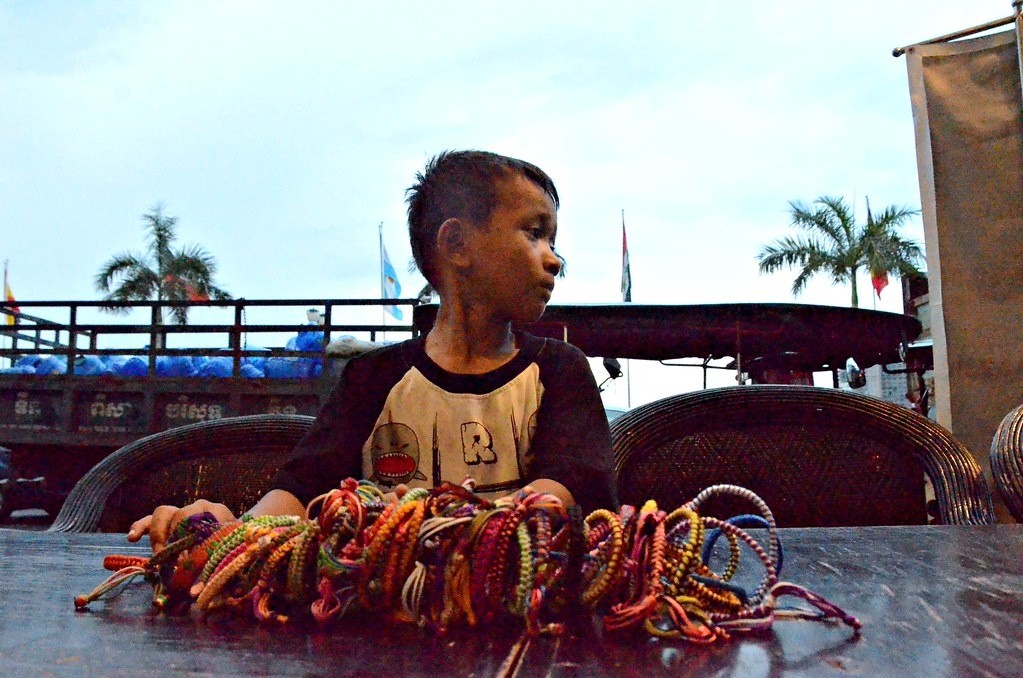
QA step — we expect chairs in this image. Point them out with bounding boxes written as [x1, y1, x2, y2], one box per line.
[991, 405, 1023, 522]
[40, 412, 318, 535]
[607, 384, 998, 528]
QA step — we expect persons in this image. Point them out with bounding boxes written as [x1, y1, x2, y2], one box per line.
[126, 148, 622, 558]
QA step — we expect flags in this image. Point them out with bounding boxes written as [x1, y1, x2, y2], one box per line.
[623, 226, 633, 304]
[870, 269, 889, 302]
[380, 241, 404, 321]
[4, 279, 22, 325]
[165, 272, 211, 307]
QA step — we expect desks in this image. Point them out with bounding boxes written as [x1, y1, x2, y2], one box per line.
[0, 529, 1023, 678]
